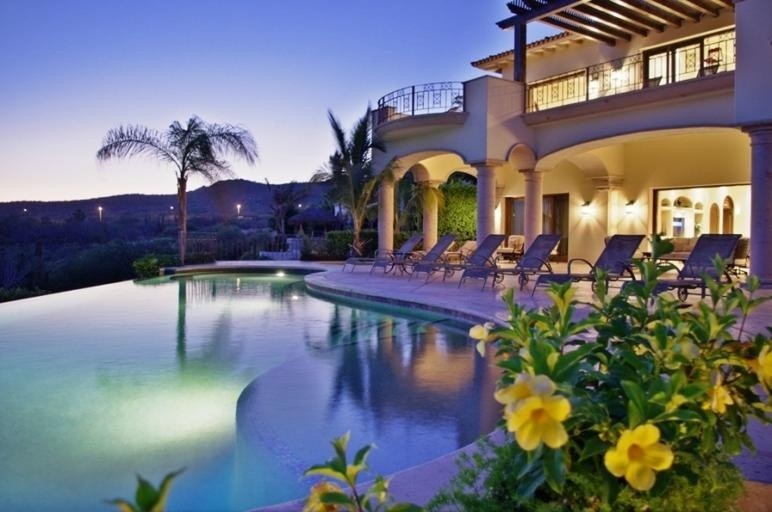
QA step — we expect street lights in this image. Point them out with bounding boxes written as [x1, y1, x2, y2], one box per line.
[237, 204, 241, 214]
[98, 206, 103, 221]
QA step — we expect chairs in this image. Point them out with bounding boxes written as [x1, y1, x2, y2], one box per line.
[644, 65, 722, 88]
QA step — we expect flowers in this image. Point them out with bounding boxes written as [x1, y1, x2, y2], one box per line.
[470, 278, 772, 511]
